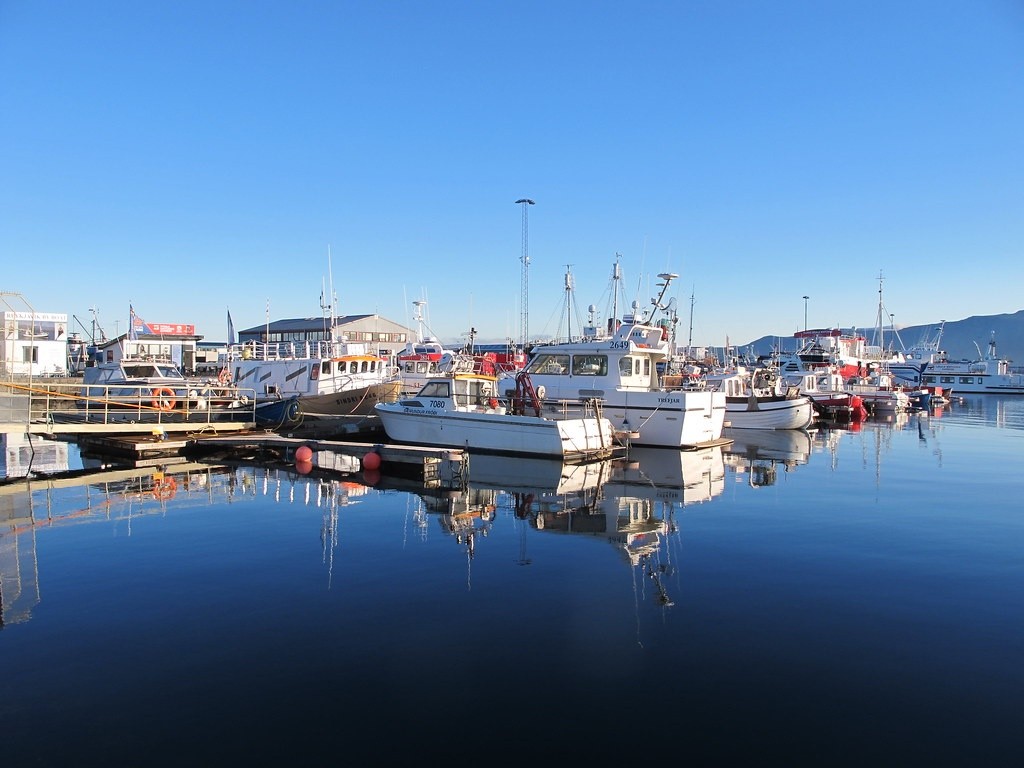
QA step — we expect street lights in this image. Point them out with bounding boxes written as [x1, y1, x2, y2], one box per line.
[803, 296, 810, 331]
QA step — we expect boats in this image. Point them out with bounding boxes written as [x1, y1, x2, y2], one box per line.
[77, 359, 229, 410]
[437, 445, 616, 535]
[374, 370, 639, 463]
[230, 251, 1023, 471]
[611, 447, 726, 504]
[485, 255, 736, 450]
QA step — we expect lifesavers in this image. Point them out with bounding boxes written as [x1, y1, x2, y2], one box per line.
[150, 388, 177, 411]
[218, 370, 230, 383]
[154, 477, 177, 501]
